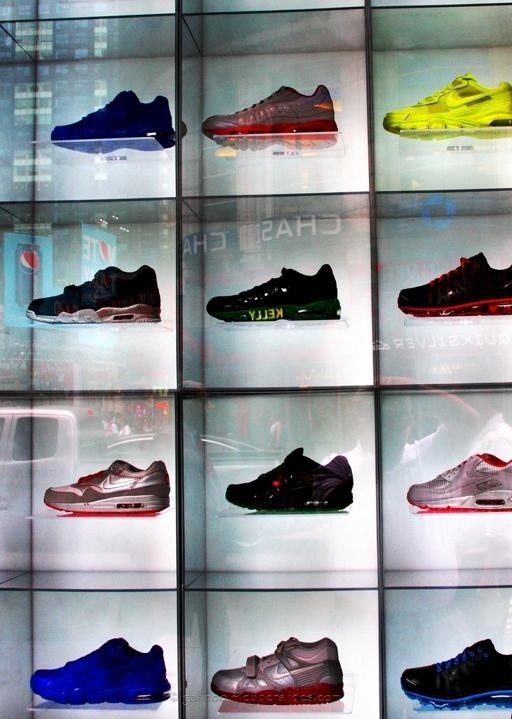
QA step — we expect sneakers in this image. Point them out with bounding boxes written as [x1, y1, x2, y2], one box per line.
[26, 265, 161, 324]
[407, 453, 511, 509]
[49, 92, 174, 154]
[211, 636, 342, 705]
[207, 263, 341, 322]
[44, 461, 168, 512]
[225, 448, 353, 511]
[383, 75, 512, 139]
[402, 638, 512, 709]
[398, 251, 512, 316]
[30, 639, 170, 705]
[202, 86, 336, 149]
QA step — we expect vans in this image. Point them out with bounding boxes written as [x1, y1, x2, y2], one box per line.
[1, 409, 78, 545]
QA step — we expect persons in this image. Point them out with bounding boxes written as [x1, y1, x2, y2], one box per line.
[268, 413, 283, 449]
[150, 377, 236, 705]
[75, 407, 154, 443]
[436, 367, 512, 642]
[322, 371, 484, 670]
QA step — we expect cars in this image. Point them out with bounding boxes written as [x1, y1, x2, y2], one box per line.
[80, 435, 278, 464]
